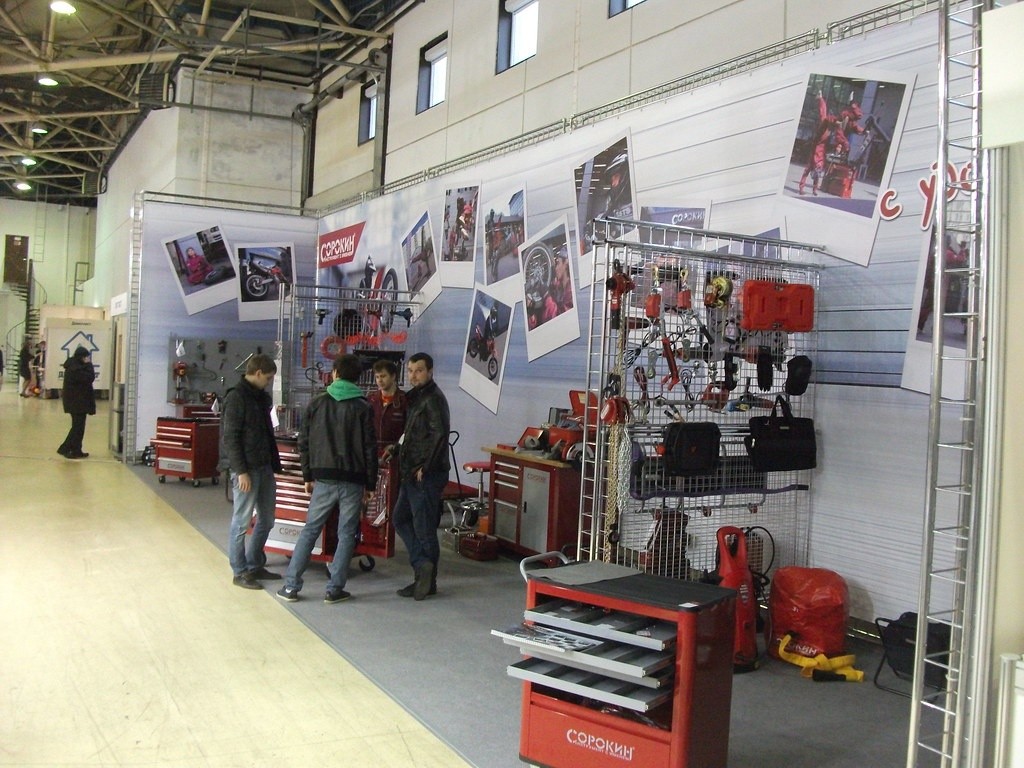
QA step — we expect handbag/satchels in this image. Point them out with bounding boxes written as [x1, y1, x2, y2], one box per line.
[661, 421, 722, 476]
[743, 395, 816, 472]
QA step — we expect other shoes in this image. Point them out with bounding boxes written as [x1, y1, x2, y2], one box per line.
[57, 447, 89, 459]
[324, 591, 350, 603]
[396, 582, 437, 597]
[240, 577, 264, 589]
[232, 576, 242, 585]
[413, 562, 433, 601]
[276, 585, 298, 601]
[247, 568, 282, 580]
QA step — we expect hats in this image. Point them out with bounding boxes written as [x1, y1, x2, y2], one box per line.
[784, 355, 813, 395]
[559, 244, 568, 259]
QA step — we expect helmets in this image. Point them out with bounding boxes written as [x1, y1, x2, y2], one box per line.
[490, 306, 497, 316]
[604, 150, 631, 209]
[364, 258, 377, 288]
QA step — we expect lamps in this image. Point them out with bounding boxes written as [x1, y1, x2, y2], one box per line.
[16, 72, 58, 191]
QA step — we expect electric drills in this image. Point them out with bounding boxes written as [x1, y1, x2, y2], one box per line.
[391, 308, 414, 329]
[740, 376, 781, 408]
[315, 309, 333, 325]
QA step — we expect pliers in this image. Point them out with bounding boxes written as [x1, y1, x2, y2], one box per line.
[664, 404, 685, 422]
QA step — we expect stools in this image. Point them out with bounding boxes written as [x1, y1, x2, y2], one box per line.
[460, 461, 491, 530]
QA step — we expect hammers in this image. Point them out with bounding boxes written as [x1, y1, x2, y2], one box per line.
[690, 311, 715, 345]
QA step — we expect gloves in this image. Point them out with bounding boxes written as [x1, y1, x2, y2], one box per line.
[756, 346, 773, 392]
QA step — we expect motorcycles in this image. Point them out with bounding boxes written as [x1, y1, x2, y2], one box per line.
[242, 253, 291, 301]
[469, 325, 498, 377]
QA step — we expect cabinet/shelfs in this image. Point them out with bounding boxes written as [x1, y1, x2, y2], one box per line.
[148, 416, 222, 487]
[244, 438, 396, 580]
[483, 448, 599, 568]
[503, 561, 738, 768]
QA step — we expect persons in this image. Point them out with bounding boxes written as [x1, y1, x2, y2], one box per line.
[56, 347, 96, 458]
[245, 256, 288, 283]
[0, 341, 46, 398]
[219, 354, 284, 590]
[410, 151, 636, 361]
[276, 354, 379, 603]
[366, 359, 410, 444]
[382, 352, 450, 602]
[184, 247, 213, 285]
[917, 225, 969, 337]
[798, 89, 870, 196]
